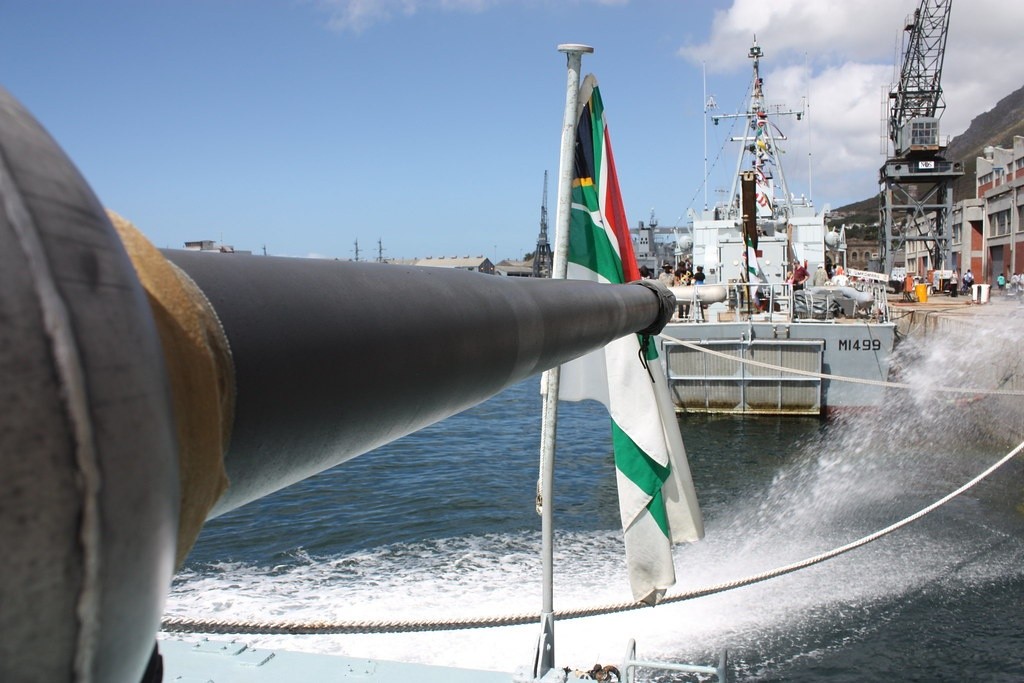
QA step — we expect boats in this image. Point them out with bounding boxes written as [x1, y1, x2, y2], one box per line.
[651, 40, 902, 418]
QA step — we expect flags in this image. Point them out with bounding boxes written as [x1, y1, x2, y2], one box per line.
[536, 45, 704, 607]
[741, 222, 761, 299]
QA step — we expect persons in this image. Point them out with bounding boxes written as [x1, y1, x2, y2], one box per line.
[950, 269, 1024, 298]
[902, 275, 930, 301]
[787, 259, 844, 299]
[640, 256, 706, 319]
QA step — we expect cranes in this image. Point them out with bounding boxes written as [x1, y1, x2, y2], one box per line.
[872, 0, 983, 299]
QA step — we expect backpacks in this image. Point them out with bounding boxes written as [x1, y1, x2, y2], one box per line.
[967, 273, 972, 283]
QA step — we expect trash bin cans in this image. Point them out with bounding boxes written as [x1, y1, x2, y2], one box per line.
[915, 284, 928, 302]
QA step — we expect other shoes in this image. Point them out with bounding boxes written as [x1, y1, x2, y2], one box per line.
[679, 314, 683, 317]
[685, 314, 688, 318]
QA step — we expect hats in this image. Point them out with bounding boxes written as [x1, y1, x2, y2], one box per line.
[662, 263, 672, 269]
[818, 265, 823, 269]
